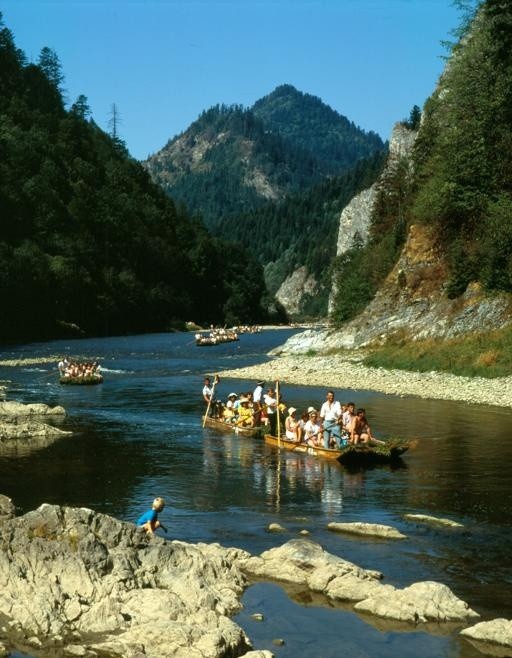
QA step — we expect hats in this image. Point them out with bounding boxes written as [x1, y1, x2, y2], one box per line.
[256, 380, 265, 385]
[307, 407, 316, 414]
[240, 398, 250, 404]
[227, 393, 238, 399]
[288, 406, 296, 416]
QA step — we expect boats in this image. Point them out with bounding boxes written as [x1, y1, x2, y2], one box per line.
[194, 325, 263, 345]
[202, 407, 285, 438]
[263, 432, 412, 463]
[59, 372, 106, 386]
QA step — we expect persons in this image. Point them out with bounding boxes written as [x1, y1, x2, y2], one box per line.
[209, 389, 372, 449]
[202, 377, 221, 418]
[57, 356, 103, 380]
[252, 379, 265, 427]
[194, 321, 260, 345]
[137, 498, 167, 536]
